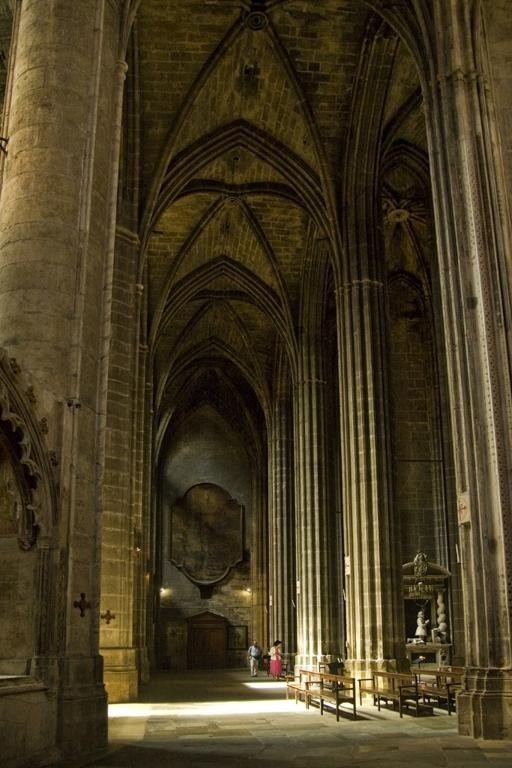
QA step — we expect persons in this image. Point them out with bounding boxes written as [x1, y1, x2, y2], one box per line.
[415, 610, 430, 640]
[268, 640, 283, 679]
[247, 639, 262, 677]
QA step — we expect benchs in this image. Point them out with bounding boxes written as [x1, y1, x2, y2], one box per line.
[286, 663, 465, 722]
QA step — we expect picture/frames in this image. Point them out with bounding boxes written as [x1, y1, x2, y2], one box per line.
[226, 625, 248, 650]
